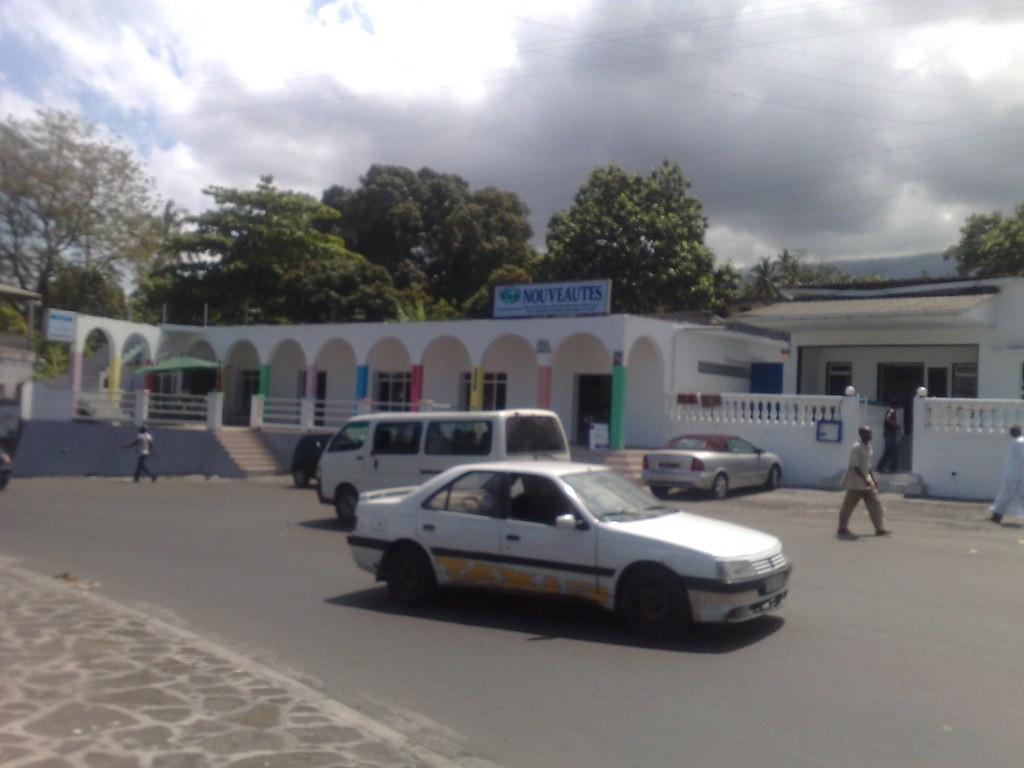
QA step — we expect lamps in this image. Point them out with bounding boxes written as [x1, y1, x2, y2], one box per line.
[917, 386, 928, 398]
[844, 384, 856, 397]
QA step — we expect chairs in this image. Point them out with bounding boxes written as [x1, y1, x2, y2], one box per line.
[511, 492, 534, 521]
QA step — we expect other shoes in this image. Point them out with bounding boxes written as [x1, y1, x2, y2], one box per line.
[838, 528, 852, 537]
[890, 470, 899, 474]
[876, 466, 886, 473]
[876, 529, 891, 535]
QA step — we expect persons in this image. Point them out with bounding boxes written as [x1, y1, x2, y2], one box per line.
[122, 423, 158, 484]
[877, 397, 901, 473]
[990, 426, 1024, 524]
[837, 425, 892, 538]
[511, 491, 587, 529]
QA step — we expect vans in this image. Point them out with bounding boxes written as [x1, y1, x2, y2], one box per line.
[314, 408, 572, 527]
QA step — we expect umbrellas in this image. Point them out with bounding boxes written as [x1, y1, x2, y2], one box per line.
[134, 351, 222, 393]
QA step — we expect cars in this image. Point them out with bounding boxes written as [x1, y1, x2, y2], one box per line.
[348, 458, 793, 634]
[641, 432, 787, 499]
[290, 427, 346, 488]
